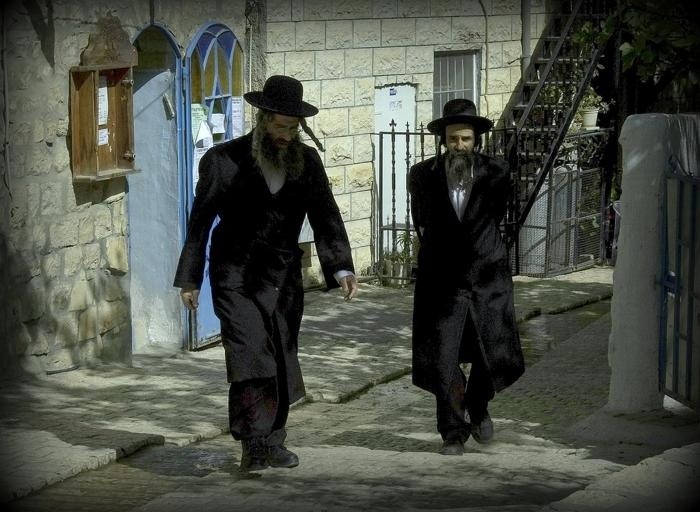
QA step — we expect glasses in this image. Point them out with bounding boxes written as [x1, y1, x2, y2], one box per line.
[276, 127, 301, 135]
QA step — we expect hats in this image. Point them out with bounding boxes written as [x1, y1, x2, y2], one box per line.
[427, 99, 494, 135]
[244, 75, 318, 117]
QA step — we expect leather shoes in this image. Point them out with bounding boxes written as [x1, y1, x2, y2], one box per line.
[441, 438, 465, 456]
[269, 445, 299, 467]
[467, 405, 494, 443]
[241, 443, 269, 470]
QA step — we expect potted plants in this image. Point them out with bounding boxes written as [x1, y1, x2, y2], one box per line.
[378, 231, 418, 289]
[576, 88, 617, 129]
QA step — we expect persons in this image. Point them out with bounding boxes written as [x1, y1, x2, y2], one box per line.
[406, 99, 525, 453]
[172, 76, 358, 474]
